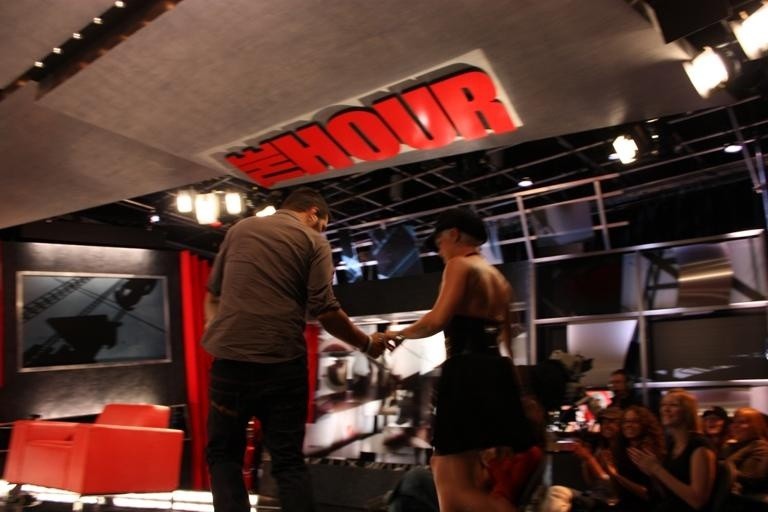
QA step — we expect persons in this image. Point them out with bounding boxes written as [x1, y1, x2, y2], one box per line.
[605, 369, 641, 406]
[606, 405, 669, 512]
[721, 406, 768, 512]
[201, 188, 384, 512]
[383, 207, 533, 512]
[627, 389, 717, 512]
[700, 405, 729, 447]
[537, 403, 625, 512]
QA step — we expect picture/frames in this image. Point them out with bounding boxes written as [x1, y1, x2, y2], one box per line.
[15, 270, 172, 374]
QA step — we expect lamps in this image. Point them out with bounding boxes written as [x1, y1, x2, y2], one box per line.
[176, 188, 192, 213]
[225, 186, 241, 215]
[729, 0, 768, 60]
[518, 169, 533, 187]
[195, 186, 218, 225]
[612, 124, 660, 166]
[254, 201, 275, 218]
[724, 135, 742, 154]
[680, 45, 742, 100]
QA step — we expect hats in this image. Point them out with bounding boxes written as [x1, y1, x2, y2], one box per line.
[701, 406, 727, 417]
[424, 207, 487, 252]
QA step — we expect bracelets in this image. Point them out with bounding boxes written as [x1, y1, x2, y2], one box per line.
[395, 331, 406, 342]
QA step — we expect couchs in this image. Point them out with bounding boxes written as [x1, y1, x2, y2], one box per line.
[1, 403, 184, 507]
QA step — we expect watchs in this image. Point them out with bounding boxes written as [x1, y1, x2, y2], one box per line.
[364, 333, 373, 354]
